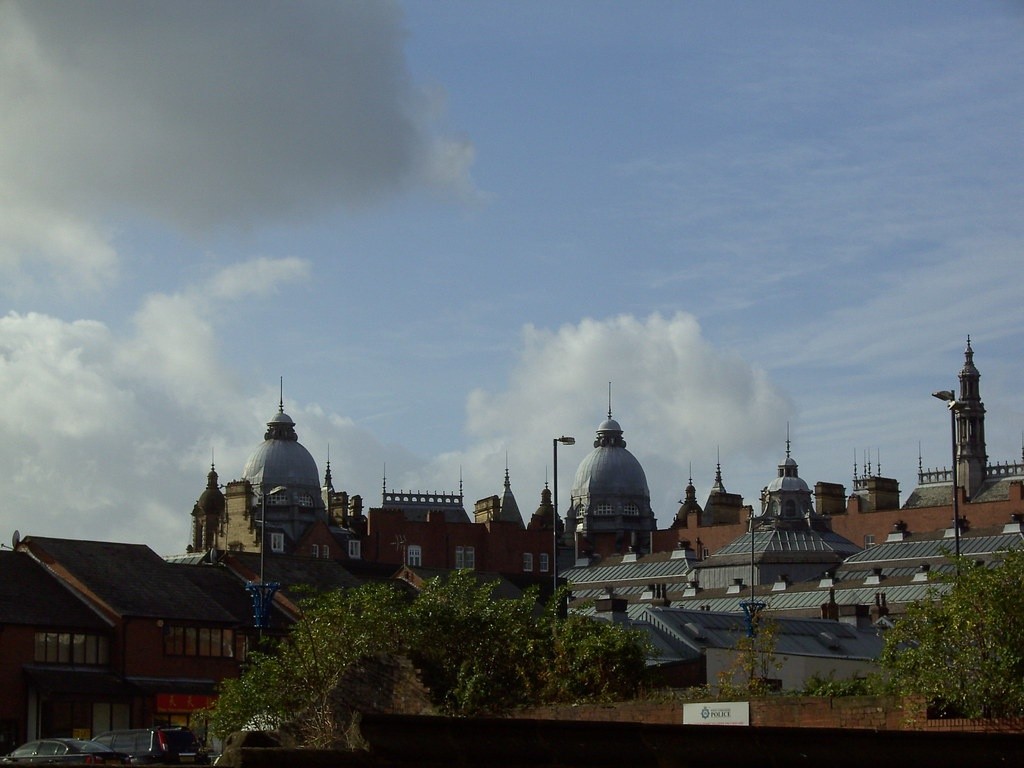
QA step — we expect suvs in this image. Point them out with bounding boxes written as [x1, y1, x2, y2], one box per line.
[80, 725, 213, 768]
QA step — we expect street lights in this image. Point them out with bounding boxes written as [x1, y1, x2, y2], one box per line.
[255, 485, 289, 653]
[551, 436, 577, 599]
[932, 390, 968, 704]
[749, 526, 774, 690]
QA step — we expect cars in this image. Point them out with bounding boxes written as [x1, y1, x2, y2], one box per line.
[0, 737, 133, 768]
